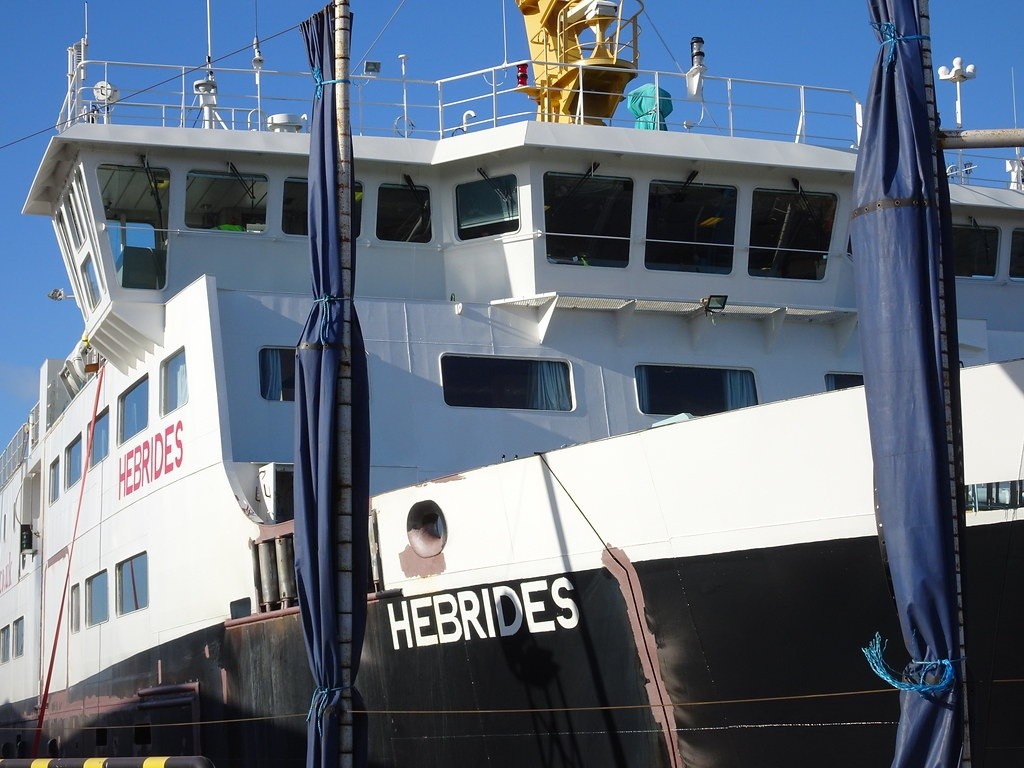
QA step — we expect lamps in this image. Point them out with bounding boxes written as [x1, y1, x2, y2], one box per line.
[700, 295, 728, 317]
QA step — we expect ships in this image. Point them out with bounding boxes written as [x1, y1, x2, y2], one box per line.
[1, 0, 1023, 767]
